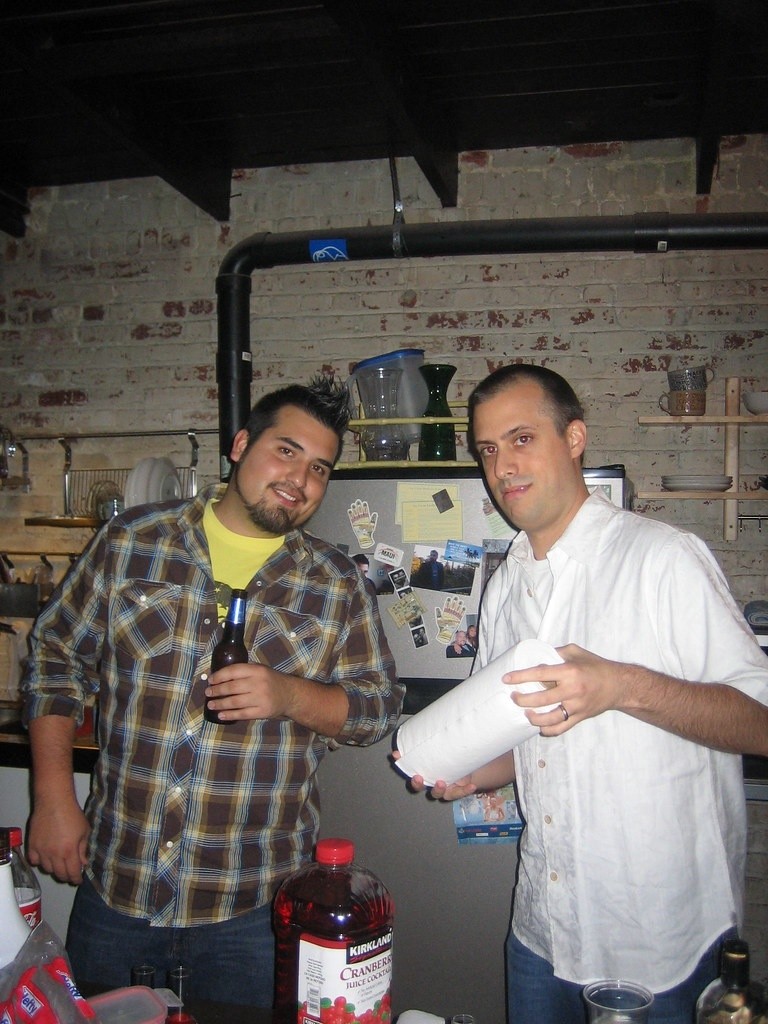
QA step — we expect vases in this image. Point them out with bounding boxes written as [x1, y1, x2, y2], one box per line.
[418, 363, 457, 461]
[358, 367, 411, 461]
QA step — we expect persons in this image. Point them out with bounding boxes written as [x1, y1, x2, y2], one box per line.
[422, 550, 445, 591]
[389, 568, 427, 648]
[17, 384, 407, 1024]
[392, 363, 768, 1024]
[447, 625, 479, 658]
[351, 554, 376, 593]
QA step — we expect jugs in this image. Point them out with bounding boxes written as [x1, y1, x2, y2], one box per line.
[345, 349, 428, 443]
[0, 425, 16, 478]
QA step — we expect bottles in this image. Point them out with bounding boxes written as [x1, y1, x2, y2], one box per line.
[273, 839, 394, 1024]
[696, 938, 768, 1024]
[166, 964, 194, 1024]
[7, 828, 42, 929]
[129, 965, 156, 990]
[204, 589, 247, 725]
[0, 827, 32, 968]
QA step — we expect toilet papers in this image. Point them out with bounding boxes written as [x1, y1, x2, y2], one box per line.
[395, 638, 566, 789]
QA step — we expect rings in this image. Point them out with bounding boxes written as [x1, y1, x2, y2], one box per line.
[558, 704, 569, 720]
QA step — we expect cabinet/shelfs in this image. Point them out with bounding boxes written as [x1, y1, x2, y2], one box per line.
[636, 376, 768, 540]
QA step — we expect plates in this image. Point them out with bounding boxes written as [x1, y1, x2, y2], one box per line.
[124, 457, 181, 511]
[661, 476, 732, 492]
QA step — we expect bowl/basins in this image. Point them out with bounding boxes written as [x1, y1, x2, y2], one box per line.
[744, 392, 768, 416]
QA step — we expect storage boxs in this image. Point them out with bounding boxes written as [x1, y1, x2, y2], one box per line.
[355, 349, 429, 444]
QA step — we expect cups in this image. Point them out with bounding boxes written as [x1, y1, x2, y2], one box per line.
[667, 365, 715, 391]
[659, 391, 705, 416]
[583, 978, 654, 1024]
